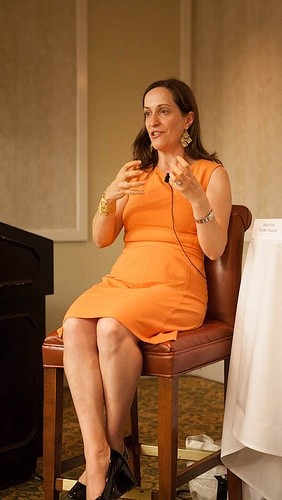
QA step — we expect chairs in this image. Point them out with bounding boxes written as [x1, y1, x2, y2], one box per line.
[42, 204, 253, 500]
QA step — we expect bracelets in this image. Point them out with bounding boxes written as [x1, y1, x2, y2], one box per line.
[97, 194, 116, 216]
[195, 208, 216, 224]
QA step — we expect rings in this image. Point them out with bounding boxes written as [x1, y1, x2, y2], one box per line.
[177, 181, 183, 186]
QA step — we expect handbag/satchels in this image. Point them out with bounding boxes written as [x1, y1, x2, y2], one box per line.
[186, 433, 229, 500]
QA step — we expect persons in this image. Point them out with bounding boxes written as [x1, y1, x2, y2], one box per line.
[57, 79, 233, 500]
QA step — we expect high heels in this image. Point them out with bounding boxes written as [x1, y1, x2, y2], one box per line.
[67, 447, 129, 500]
[96, 448, 137, 500]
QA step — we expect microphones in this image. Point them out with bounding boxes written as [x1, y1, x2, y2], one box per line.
[165, 173, 170, 183]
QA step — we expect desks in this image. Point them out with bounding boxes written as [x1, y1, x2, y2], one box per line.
[217, 219, 282, 500]
